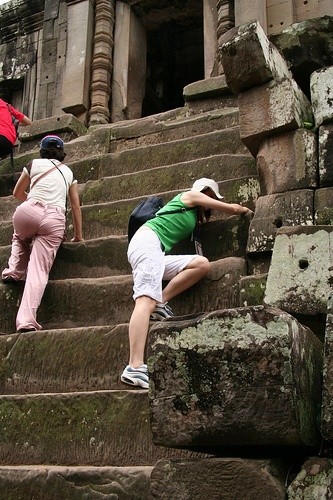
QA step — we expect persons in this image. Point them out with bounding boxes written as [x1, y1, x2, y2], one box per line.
[121, 177, 255, 387]
[0, 99, 31, 158]
[1, 135, 84, 332]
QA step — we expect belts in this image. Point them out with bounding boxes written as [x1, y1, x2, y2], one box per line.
[25, 197, 65, 215]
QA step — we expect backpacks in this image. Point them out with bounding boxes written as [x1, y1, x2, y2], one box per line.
[127, 190, 200, 243]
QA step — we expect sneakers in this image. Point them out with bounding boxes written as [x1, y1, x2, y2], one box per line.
[149, 301, 174, 321]
[120, 364, 149, 388]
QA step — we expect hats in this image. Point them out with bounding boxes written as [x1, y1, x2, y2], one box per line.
[40, 135, 63, 147]
[191, 178, 225, 200]
